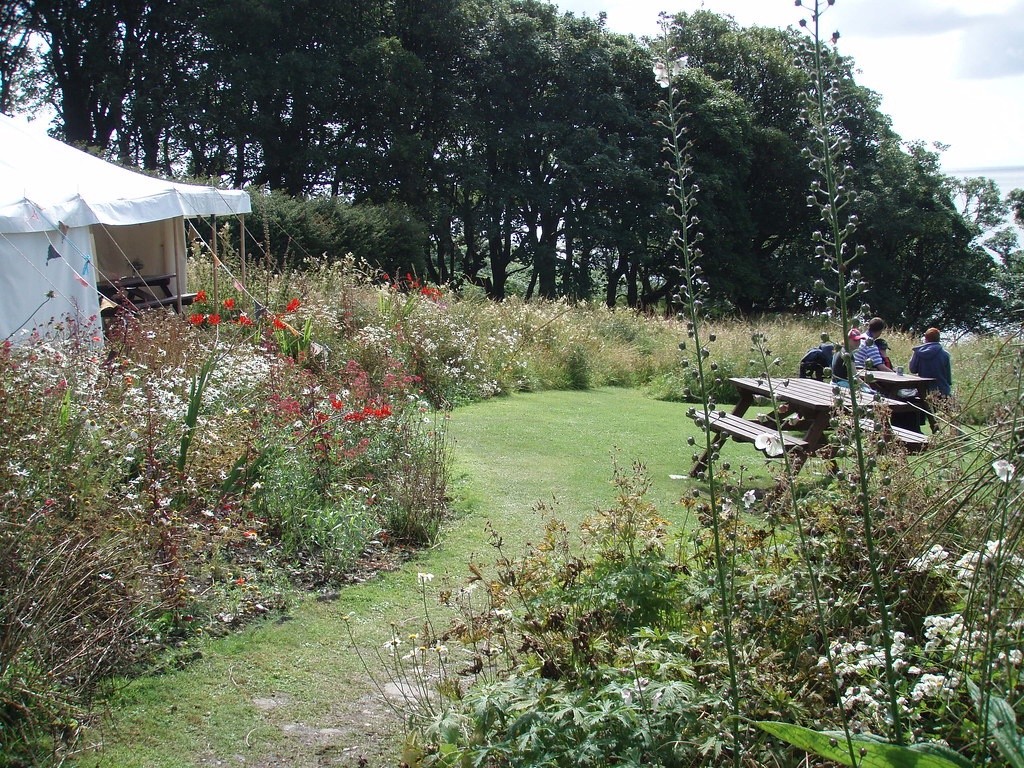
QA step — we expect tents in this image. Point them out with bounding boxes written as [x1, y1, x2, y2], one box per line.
[0, 112, 250, 346]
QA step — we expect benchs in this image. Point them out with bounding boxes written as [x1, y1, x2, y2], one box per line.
[126, 293, 200, 310]
[686, 409, 933, 458]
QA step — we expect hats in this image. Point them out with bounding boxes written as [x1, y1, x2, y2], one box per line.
[874, 339, 891, 350]
[848, 328, 866, 340]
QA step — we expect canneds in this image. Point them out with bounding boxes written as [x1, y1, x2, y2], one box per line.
[896, 366, 903, 376]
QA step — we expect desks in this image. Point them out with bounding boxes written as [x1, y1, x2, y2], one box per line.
[690, 378, 908, 512]
[96, 276, 192, 328]
[856, 369, 943, 439]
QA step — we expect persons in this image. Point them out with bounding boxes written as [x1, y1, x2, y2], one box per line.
[854, 318, 894, 394]
[800, 344, 833, 381]
[899, 327, 952, 398]
[832, 328, 871, 392]
